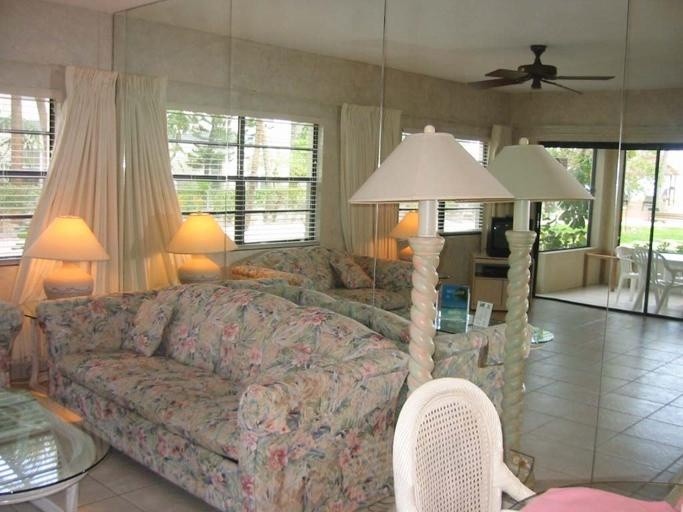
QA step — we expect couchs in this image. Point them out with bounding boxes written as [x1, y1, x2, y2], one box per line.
[227, 245, 413, 319]
[230, 280, 537, 422]
[32, 283, 409, 511]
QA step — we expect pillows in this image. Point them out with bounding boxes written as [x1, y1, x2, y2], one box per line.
[328, 252, 376, 291]
[119, 298, 173, 360]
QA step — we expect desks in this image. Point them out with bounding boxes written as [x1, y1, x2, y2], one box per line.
[587, 249, 620, 292]
[504, 481, 683, 512]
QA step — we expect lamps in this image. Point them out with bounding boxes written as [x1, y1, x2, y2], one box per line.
[486, 134, 597, 471]
[166, 212, 238, 286]
[347, 124, 515, 402]
[23, 216, 112, 299]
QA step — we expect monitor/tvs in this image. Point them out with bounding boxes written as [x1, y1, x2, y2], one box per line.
[487, 217, 533, 259]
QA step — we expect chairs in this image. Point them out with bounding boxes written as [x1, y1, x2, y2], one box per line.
[393, 376, 539, 512]
[614, 244, 683, 314]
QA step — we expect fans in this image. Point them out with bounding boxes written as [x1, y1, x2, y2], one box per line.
[469, 46, 615, 95]
